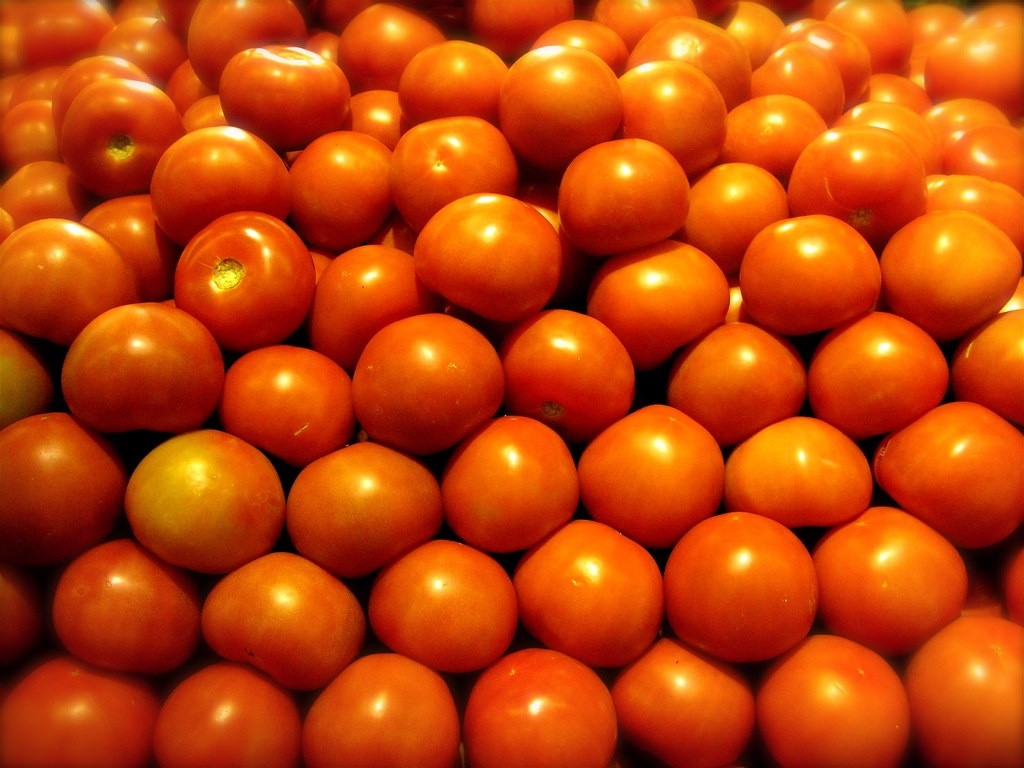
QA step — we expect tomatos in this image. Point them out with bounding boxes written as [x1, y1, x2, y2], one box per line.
[0, 0, 1024, 768]
